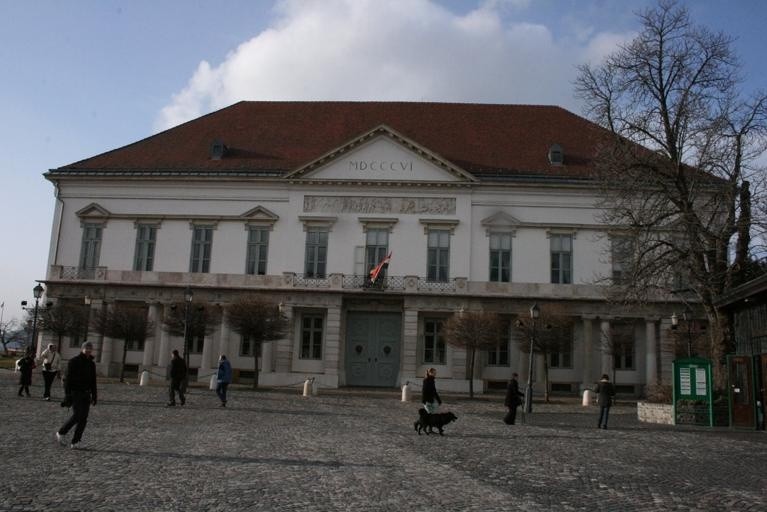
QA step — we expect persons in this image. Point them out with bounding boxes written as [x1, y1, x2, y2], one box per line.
[216, 354, 232, 407]
[503, 373, 524, 425]
[167, 350, 186, 405]
[592, 375, 616, 429]
[18, 351, 34, 396]
[422, 368, 441, 433]
[56, 341, 97, 449]
[40, 343, 62, 400]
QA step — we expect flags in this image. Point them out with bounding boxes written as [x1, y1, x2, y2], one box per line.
[370, 252, 392, 283]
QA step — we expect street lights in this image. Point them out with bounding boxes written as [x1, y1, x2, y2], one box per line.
[182, 285, 196, 376]
[29, 284, 45, 366]
[522, 300, 541, 414]
[683, 302, 695, 359]
[670, 310, 680, 359]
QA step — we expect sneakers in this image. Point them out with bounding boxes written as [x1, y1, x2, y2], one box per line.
[70, 440, 88, 450]
[56, 431, 69, 449]
[18, 392, 51, 401]
[164, 399, 230, 408]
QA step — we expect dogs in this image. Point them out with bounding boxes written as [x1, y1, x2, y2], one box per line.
[418, 408, 458, 436]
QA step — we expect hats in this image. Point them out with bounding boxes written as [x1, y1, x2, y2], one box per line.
[80, 340, 92, 351]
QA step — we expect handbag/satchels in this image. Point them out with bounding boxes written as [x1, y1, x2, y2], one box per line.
[44, 362, 52, 371]
